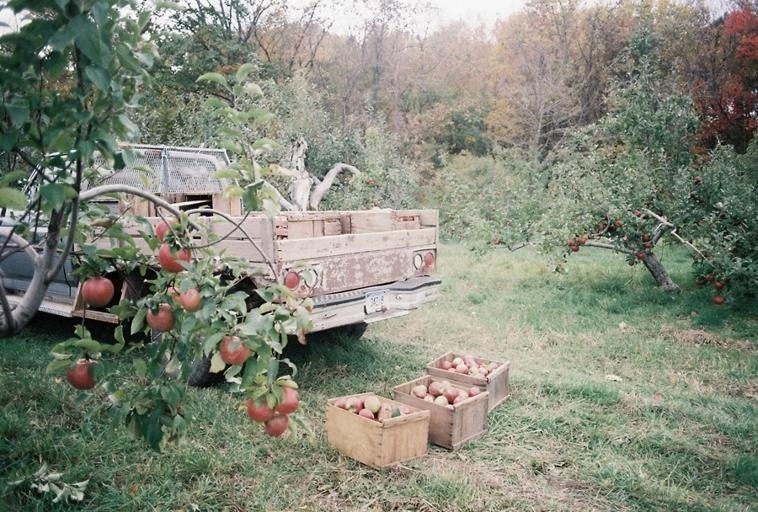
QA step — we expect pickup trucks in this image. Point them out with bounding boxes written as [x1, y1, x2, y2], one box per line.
[1, 144, 442, 387]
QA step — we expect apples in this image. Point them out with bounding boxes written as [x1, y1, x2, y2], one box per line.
[180, 288, 201, 312]
[159, 244, 191, 273]
[82, 276, 114, 307]
[330, 395, 411, 423]
[146, 303, 174, 332]
[247, 387, 300, 436]
[70, 359, 98, 390]
[168, 287, 184, 309]
[411, 380, 480, 409]
[220, 336, 249, 365]
[284, 272, 299, 288]
[570, 210, 726, 304]
[441, 354, 498, 378]
[156, 220, 179, 242]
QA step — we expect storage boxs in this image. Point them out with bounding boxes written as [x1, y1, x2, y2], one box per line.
[116, 188, 423, 241]
[325, 349, 511, 471]
